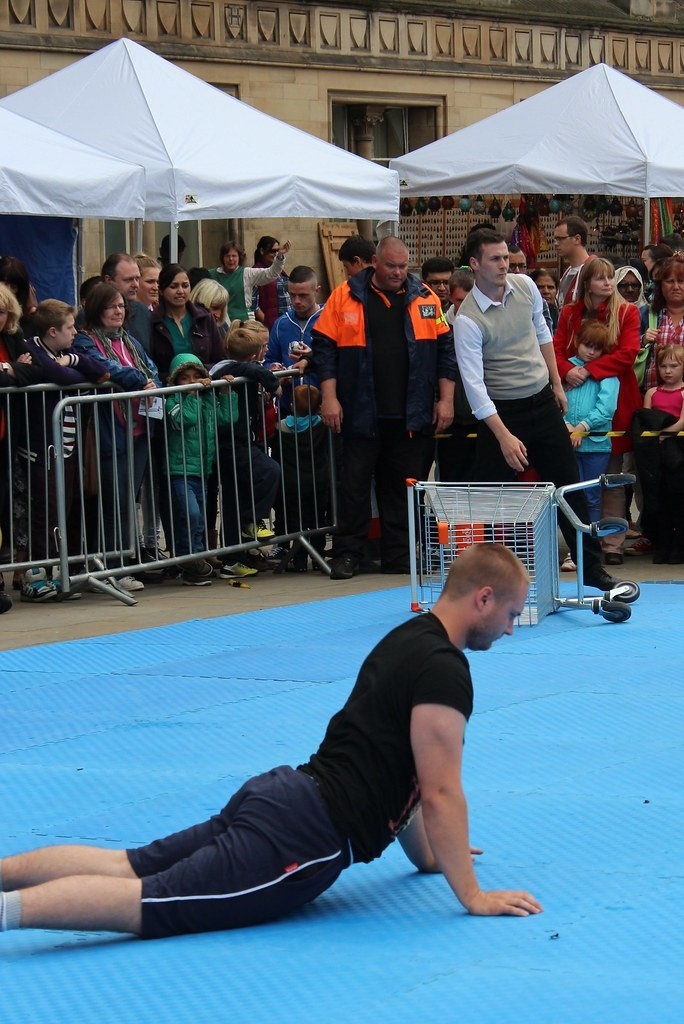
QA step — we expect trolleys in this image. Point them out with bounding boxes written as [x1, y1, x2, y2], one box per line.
[404, 472, 641, 627]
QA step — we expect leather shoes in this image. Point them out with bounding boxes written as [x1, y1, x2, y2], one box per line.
[583, 567, 624, 591]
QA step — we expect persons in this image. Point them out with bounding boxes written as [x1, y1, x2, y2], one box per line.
[1, 540, 542, 938]
[1, 218, 683, 615]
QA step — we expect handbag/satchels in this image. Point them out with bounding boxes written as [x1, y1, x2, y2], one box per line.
[633, 303, 659, 388]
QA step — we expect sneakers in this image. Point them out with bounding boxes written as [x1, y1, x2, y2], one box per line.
[266, 545, 294, 564]
[88, 574, 146, 594]
[329, 555, 361, 579]
[51, 565, 83, 599]
[380, 553, 437, 575]
[174, 553, 213, 586]
[242, 518, 275, 539]
[624, 537, 655, 556]
[219, 561, 259, 579]
[19, 566, 58, 603]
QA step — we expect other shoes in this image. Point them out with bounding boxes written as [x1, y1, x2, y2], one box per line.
[605, 553, 624, 564]
[625, 528, 641, 538]
[560, 552, 576, 571]
[0, 590, 13, 615]
[135, 547, 169, 574]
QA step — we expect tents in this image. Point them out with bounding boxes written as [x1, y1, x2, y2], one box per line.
[388, 64, 683, 248]
[0, 38, 399, 264]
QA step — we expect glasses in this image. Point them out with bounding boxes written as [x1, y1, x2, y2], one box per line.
[553, 235, 570, 242]
[270, 248, 280, 254]
[619, 282, 642, 290]
[507, 264, 528, 273]
[424, 279, 449, 287]
